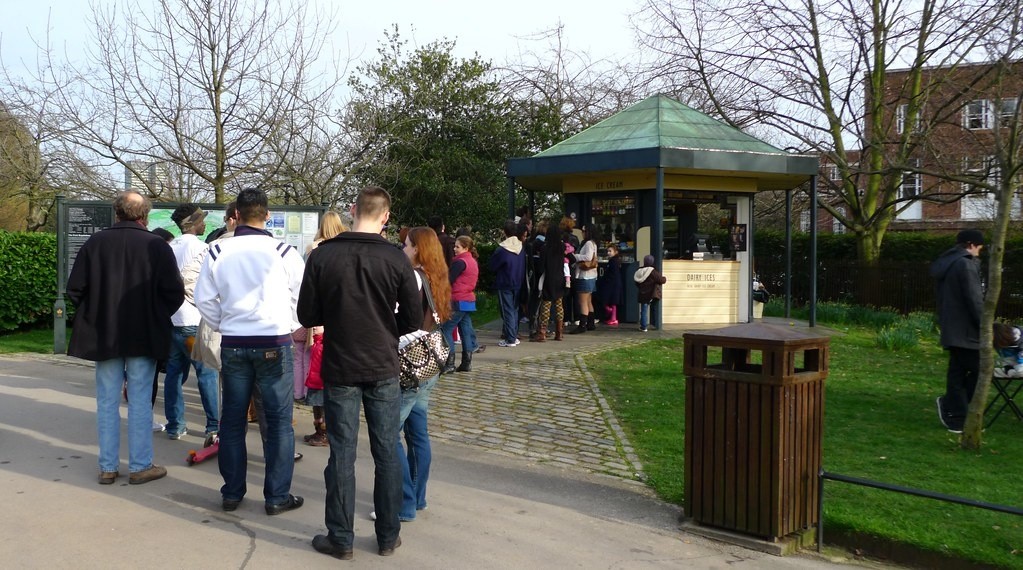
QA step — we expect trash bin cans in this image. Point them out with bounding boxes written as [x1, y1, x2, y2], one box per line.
[681, 320, 831, 542]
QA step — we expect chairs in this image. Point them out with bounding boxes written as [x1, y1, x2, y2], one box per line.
[983, 342, 1023, 425]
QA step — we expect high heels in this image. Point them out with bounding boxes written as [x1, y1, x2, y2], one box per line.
[475, 344, 487, 353]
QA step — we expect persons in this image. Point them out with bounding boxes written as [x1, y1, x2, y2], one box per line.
[602, 245, 622, 324]
[634, 255, 666, 331]
[298, 187, 425, 561]
[438, 229, 486, 373]
[66, 193, 186, 483]
[935, 231, 984, 433]
[121, 204, 342, 459]
[490, 210, 599, 346]
[371, 227, 451, 520]
[193, 189, 305, 514]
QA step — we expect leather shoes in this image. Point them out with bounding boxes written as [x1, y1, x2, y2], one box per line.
[379, 536, 401, 556]
[312, 534, 353, 560]
[222, 498, 239, 512]
[264, 494, 304, 516]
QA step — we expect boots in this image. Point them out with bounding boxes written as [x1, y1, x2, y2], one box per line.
[554, 321, 563, 340]
[441, 352, 456, 375]
[309, 423, 330, 446]
[456, 350, 473, 372]
[304, 418, 324, 441]
[529, 324, 547, 342]
[571, 313, 589, 334]
[585, 311, 596, 331]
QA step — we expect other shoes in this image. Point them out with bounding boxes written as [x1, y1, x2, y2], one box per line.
[292, 419, 296, 424]
[203, 431, 218, 449]
[370, 511, 410, 522]
[936, 396, 952, 427]
[499, 311, 648, 339]
[170, 426, 188, 440]
[152, 421, 165, 431]
[947, 421, 986, 433]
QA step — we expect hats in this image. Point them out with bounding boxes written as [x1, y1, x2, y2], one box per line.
[178, 207, 209, 233]
[644, 255, 654, 265]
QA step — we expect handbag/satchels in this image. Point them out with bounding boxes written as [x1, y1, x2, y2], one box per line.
[399, 328, 452, 390]
[579, 240, 597, 271]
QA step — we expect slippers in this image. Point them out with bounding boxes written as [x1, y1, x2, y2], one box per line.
[293, 452, 303, 460]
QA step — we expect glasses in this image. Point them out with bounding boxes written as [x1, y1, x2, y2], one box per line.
[582, 230, 585, 234]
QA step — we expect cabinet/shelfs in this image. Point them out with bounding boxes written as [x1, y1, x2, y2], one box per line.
[588, 195, 637, 249]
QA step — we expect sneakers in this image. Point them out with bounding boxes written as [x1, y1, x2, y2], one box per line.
[129, 463, 167, 485]
[98, 469, 119, 484]
[497, 338, 520, 347]
